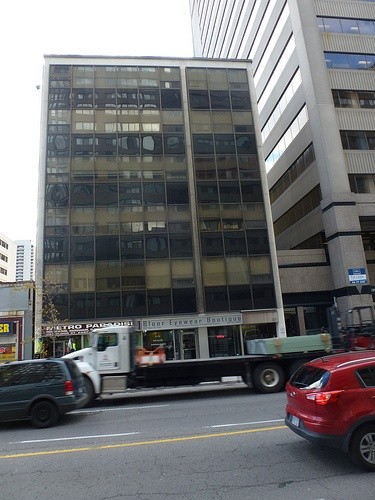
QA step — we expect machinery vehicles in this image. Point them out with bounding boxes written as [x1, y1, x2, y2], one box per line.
[327, 305, 375, 354]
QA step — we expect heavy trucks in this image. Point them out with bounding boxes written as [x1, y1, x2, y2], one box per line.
[59, 325, 339, 407]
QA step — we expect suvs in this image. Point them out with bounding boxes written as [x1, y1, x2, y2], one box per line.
[284, 350, 375, 472]
[0, 358, 89, 430]
